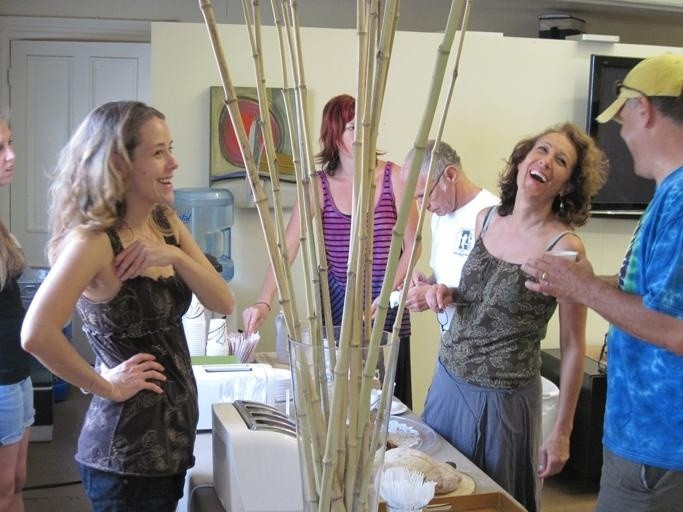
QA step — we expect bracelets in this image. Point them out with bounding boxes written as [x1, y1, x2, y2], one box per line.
[256, 301, 272, 309]
[81, 368, 98, 395]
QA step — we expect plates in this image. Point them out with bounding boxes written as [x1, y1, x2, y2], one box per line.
[390, 400, 408, 415]
[387, 415, 436, 456]
[435, 471, 475, 498]
[272, 367, 293, 403]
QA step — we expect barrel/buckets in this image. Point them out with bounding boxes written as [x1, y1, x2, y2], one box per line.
[173, 187, 234, 283]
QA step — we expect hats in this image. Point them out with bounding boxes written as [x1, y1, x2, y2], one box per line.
[594, 51, 683, 125]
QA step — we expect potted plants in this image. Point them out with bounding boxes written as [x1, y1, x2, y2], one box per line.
[196, 0, 475, 512]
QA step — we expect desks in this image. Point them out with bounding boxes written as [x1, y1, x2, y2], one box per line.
[179, 352, 529, 512]
[539, 342, 606, 493]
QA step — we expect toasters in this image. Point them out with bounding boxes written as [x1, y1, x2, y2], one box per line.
[212, 399, 308, 511]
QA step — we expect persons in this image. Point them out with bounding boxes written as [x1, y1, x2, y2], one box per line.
[20, 101, 235, 511]
[243, 91, 423, 411]
[397, 139, 502, 332]
[423, 121, 612, 511]
[521, 53, 682, 511]
[0, 108, 31, 511]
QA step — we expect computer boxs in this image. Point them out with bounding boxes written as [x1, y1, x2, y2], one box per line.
[542, 345, 612, 496]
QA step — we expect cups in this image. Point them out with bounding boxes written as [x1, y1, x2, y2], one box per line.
[206, 318, 230, 357]
[183, 293, 206, 357]
[370, 391, 381, 409]
[548, 250, 578, 261]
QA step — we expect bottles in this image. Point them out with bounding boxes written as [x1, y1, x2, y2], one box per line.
[275, 309, 289, 363]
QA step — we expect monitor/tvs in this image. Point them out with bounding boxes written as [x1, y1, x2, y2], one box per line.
[581, 50, 683, 217]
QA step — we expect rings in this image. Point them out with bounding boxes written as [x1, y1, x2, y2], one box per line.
[541, 272, 547, 280]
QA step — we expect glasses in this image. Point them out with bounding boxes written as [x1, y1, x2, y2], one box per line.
[436, 298, 488, 348]
[414, 169, 444, 199]
[610, 77, 651, 101]
